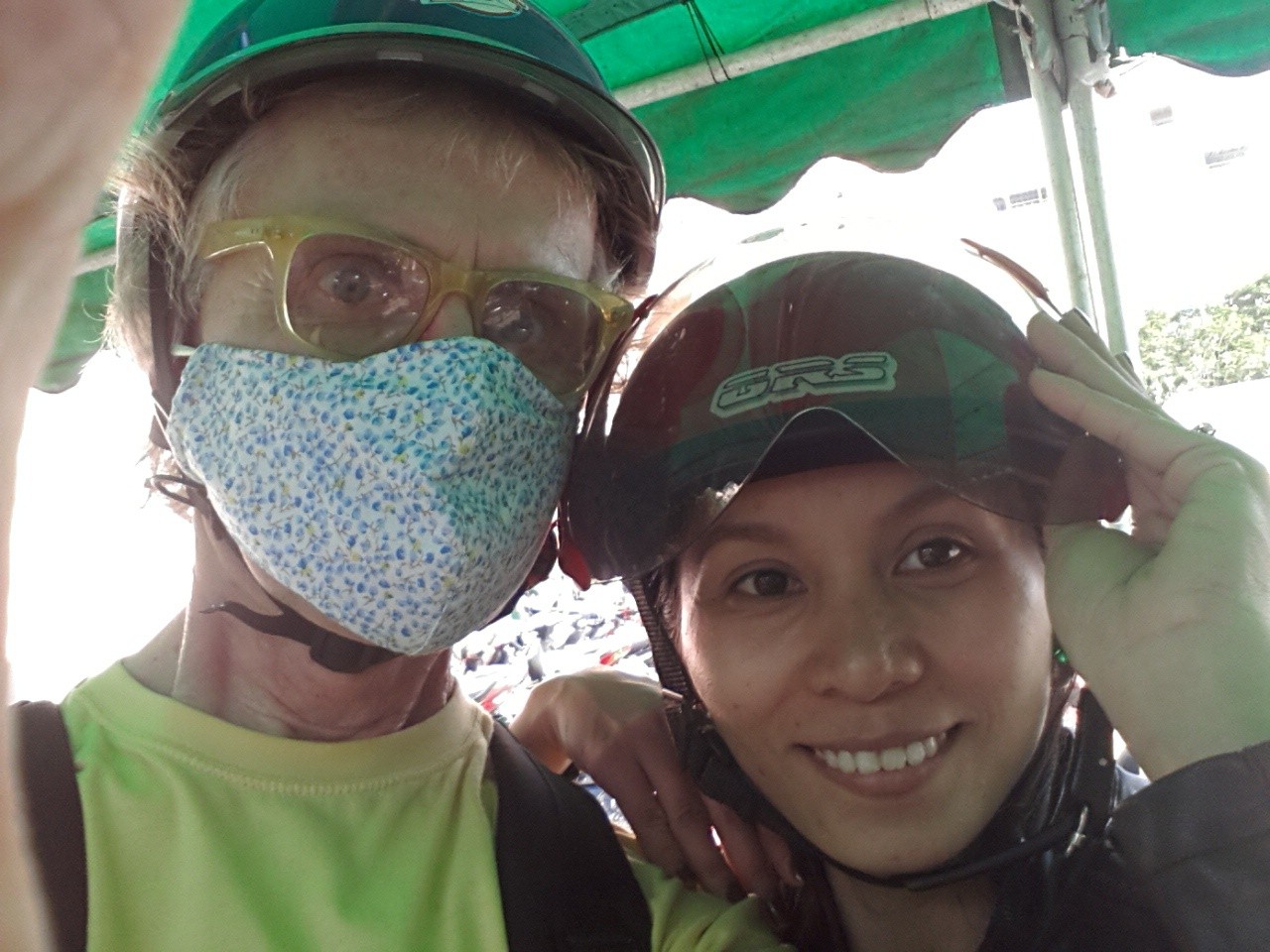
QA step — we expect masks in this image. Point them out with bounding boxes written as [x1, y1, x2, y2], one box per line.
[152, 345, 584, 653]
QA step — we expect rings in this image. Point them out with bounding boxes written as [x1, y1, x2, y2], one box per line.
[1190, 423, 1215, 440]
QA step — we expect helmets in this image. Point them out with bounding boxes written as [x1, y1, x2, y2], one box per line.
[112, 1, 664, 298]
[555, 222, 1136, 593]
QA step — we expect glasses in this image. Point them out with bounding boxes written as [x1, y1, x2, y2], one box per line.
[178, 215, 636, 401]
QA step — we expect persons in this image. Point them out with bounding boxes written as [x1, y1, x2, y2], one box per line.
[506, 218, 1270, 952]
[0, 0, 792, 952]
